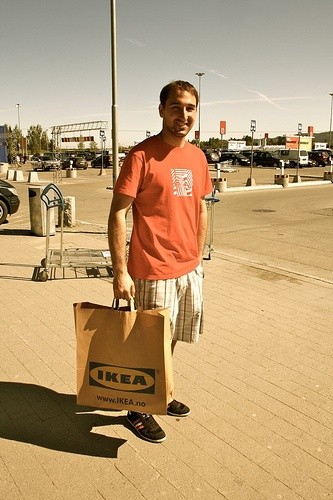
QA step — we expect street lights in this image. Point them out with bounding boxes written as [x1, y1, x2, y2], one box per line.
[16, 103, 22, 131]
[292, 123, 302, 183]
[246, 119, 258, 186]
[195, 72, 206, 149]
[328, 92, 333, 150]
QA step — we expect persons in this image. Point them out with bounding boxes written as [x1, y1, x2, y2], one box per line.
[108, 80, 214, 445]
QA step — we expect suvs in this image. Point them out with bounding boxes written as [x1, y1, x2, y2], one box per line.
[31, 155, 60, 172]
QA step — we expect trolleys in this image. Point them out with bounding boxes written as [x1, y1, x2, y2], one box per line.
[32, 183, 126, 281]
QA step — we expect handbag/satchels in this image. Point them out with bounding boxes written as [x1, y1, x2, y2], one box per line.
[72, 296, 173, 416]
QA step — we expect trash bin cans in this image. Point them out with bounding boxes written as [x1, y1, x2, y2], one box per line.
[0, 162, 8, 174]
[28, 186, 56, 236]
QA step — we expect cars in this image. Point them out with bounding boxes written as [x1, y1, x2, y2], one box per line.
[205, 153, 220, 163]
[91, 153, 114, 168]
[221, 152, 251, 166]
[239, 148, 333, 168]
[62, 156, 89, 170]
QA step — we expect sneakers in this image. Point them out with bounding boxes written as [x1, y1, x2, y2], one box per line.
[166, 399, 190, 416]
[126, 410, 166, 442]
